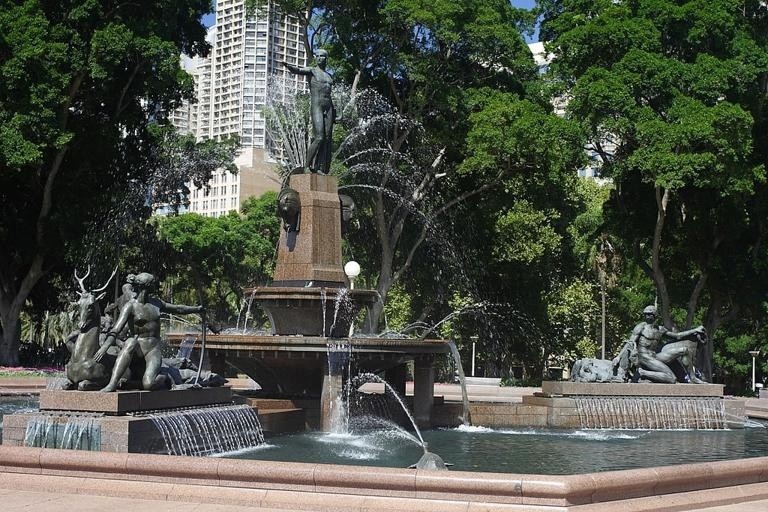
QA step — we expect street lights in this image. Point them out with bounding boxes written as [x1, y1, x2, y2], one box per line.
[470, 336, 479, 376]
[749, 351, 760, 392]
[601, 240, 613, 360]
[345, 261, 361, 338]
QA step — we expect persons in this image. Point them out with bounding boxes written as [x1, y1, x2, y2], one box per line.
[93, 269, 208, 387]
[613, 303, 708, 383]
[104, 282, 138, 313]
[281, 46, 339, 173]
